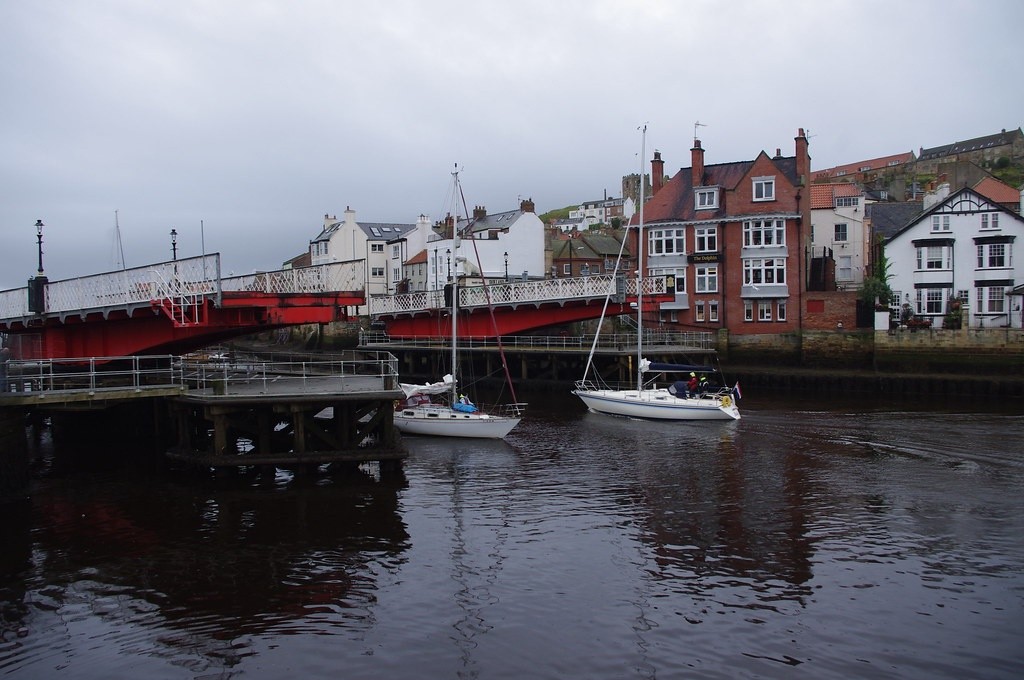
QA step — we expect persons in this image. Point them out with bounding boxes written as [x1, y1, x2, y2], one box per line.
[687, 371, 710, 399]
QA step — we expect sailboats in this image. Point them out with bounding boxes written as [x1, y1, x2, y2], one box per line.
[365, 163, 528, 440]
[569, 118, 741, 421]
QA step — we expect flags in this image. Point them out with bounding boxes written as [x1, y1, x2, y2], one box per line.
[733, 382, 742, 401]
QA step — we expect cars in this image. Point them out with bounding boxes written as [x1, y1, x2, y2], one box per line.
[207, 351, 230, 362]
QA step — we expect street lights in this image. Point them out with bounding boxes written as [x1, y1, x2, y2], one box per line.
[33, 219, 46, 315]
[169, 228, 181, 294]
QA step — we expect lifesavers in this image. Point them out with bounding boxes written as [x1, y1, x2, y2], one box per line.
[722, 395, 732, 408]
